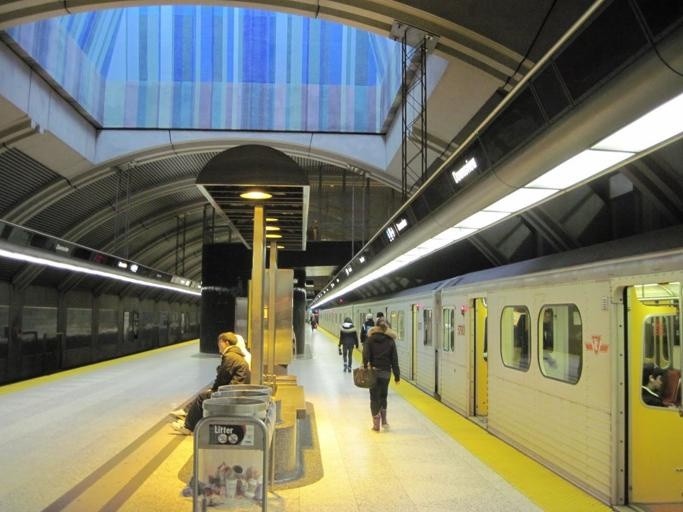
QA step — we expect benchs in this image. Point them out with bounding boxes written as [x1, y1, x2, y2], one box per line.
[275, 376, 305, 484]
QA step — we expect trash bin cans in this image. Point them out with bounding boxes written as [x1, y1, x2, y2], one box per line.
[201, 383, 274, 419]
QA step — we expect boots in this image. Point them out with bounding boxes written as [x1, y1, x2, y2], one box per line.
[344, 364, 347, 372]
[380, 408, 390, 427]
[371, 415, 380, 431]
[348, 365, 351, 372]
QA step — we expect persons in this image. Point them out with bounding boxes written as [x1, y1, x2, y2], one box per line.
[338, 317, 358, 373]
[361, 312, 393, 351]
[543, 308, 553, 350]
[362, 318, 400, 432]
[169, 331, 250, 435]
[309, 310, 319, 331]
[643, 366, 674, 407]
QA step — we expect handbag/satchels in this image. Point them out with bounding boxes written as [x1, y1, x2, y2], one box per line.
[339, 345, 342, 355]
[353, 366, 379, 387]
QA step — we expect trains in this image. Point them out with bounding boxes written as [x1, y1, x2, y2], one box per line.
[316, 223, 682, 512]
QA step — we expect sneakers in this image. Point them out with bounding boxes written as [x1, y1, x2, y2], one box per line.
[168, 408, 191, 435]
